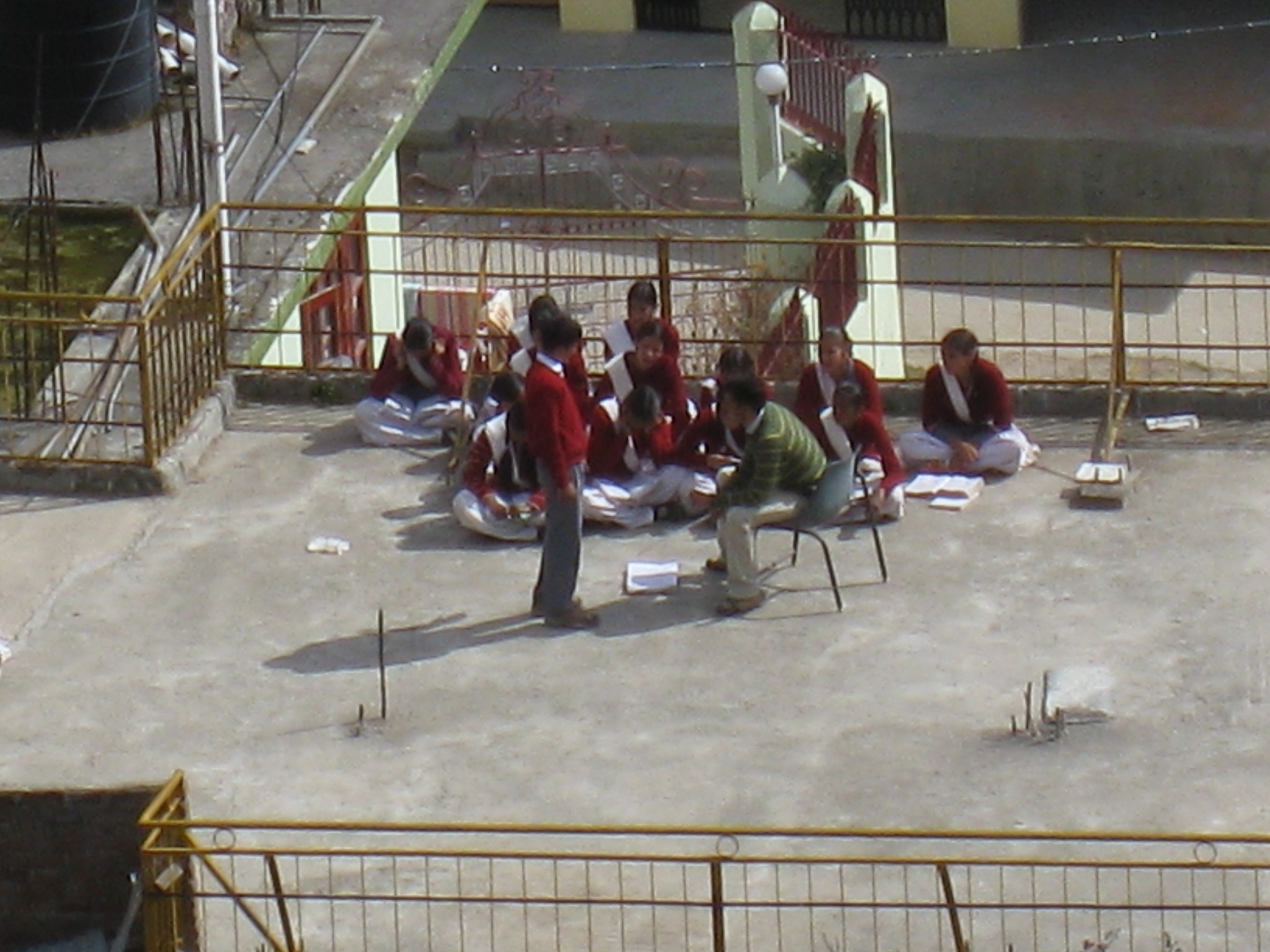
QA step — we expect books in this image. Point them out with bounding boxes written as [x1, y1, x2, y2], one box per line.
[384, 391, 865, 596]
[904, 473, 985, 512]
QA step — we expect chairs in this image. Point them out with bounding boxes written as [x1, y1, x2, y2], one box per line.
[751, 445, 887, 611]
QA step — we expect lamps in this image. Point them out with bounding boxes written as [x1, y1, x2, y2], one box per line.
[754, 63, 789, 105]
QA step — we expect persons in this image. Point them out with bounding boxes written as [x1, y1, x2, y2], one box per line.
[354, 316, 478, 448]
[706, 366, 827, 613]
[898, 328, 1034, 478]
[526, 311, 599, 629]
[451, 279, 908, 542]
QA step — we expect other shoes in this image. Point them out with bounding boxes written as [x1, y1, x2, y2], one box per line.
[706, 556, 727, 571]
[718, 587, 766, 616]
[530, 595, 582, 618]
[543, 603, 598, 629]
[655, 501, 686, 522]
[914, 457, 954, 475]
